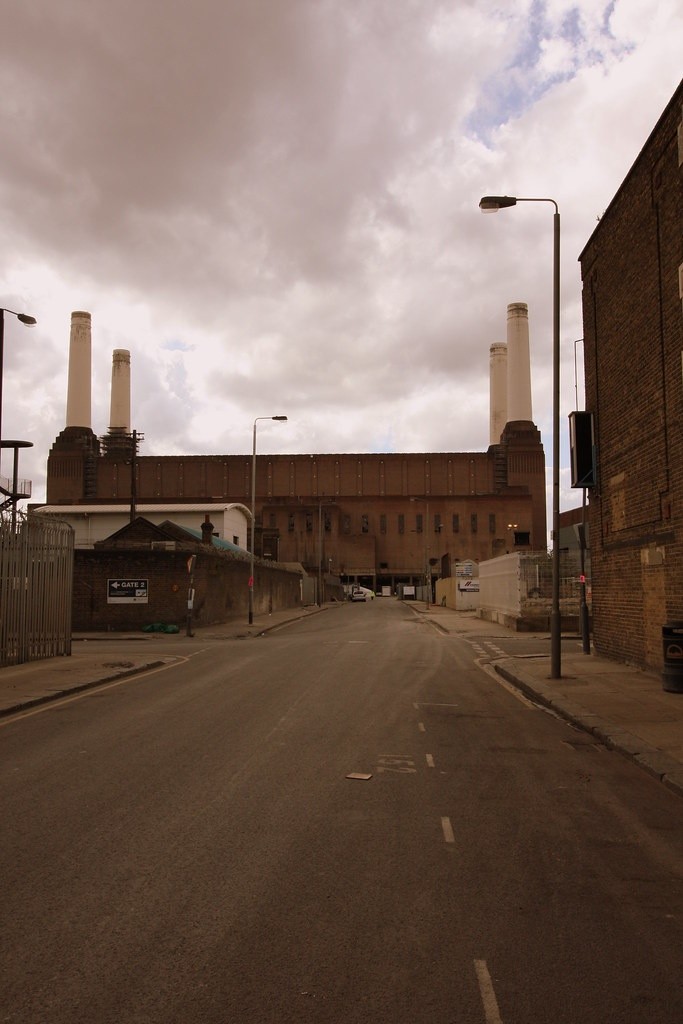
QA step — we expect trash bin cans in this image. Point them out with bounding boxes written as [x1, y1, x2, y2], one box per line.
[662, 621, 683, 693]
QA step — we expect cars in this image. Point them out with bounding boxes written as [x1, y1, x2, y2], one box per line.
[352, 590, 366, 602]
[344, 584, 375, 600]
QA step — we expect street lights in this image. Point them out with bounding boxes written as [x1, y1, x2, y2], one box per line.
[319, 499, 337, 607]
[478, 196, 560, 679]
[0, 308, 36, 467]
[410, 496, 430, 609]
[249, 416, 288, 624]
